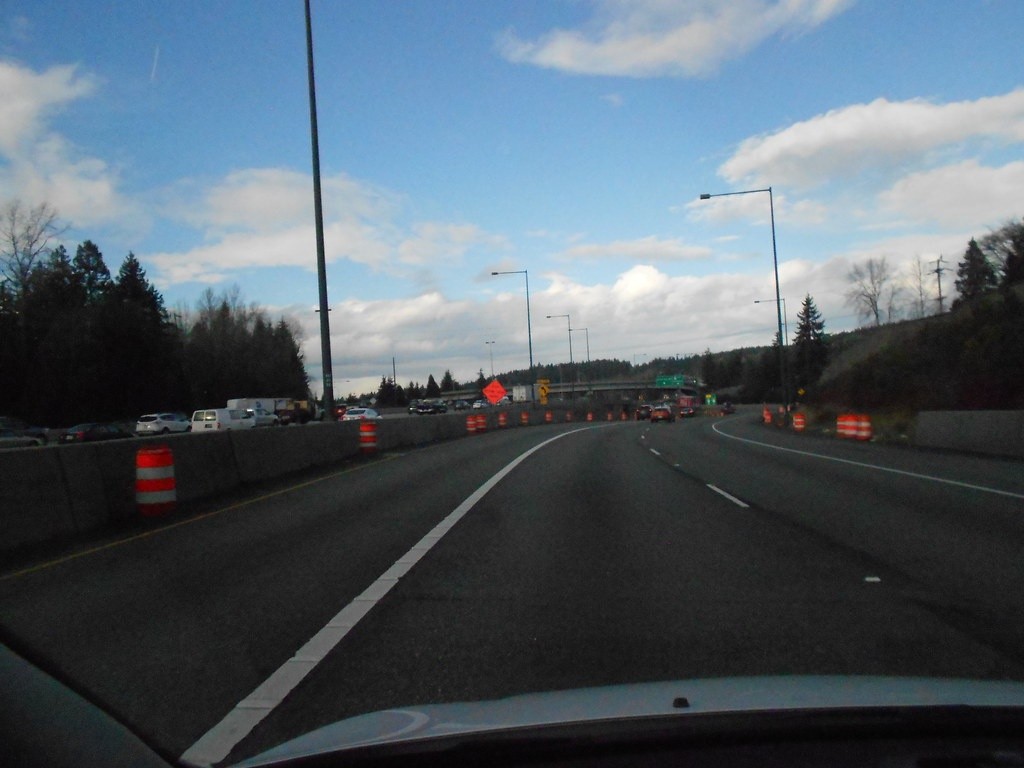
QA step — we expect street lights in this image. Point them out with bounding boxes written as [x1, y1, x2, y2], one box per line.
[547, 315, 575, 401]
[492, 269, 535, 405]
[568, 328, 592, 396]
[754, 298, 788, 347]
[486, 340, 495, 375]
[700, 187, 789, 430]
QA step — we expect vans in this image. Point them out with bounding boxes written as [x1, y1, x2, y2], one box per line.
[191, 409, 256, 432]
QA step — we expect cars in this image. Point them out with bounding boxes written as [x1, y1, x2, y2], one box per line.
[454, 396, 513, 409]
[335, 403, 384, 421]
[636, 403, 696, 423]
[409, 399, 447, 414]
[57, 423, 134, 443]
[0, 428, 44, 451]
[136, 413, 191, 435]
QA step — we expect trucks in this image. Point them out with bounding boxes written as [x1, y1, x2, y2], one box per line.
[226, 398, 322, 425]
